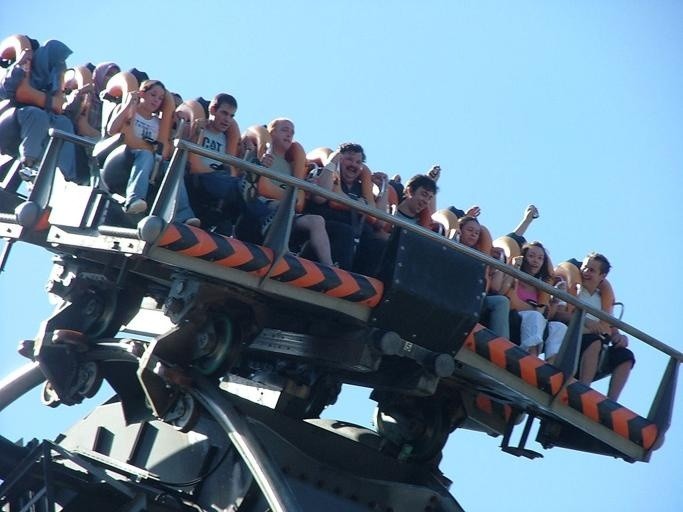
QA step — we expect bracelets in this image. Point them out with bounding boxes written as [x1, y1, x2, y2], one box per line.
[326, 161, 337, 172]
[44, 94, 53, 110]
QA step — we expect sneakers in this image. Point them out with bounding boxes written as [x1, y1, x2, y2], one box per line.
[125, 200, 147, 214]
[19, 166, 37, 184]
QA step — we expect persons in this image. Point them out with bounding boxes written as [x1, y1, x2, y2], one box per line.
[106, 79, 201, 226]
[189, 93, 265, 236]
[442, 206, 510, 338]
[465, 206, 483, 216]
[503, 238, 570, 367]
[390, 162, 456, 226]
[77, 62, 120, 137]
[256, 117, 340, 270]
[549, 253, 614, 386]
[1, 43, 86, 179]
[505, 204, 539, 245]
[310, 142, 392, 279]
